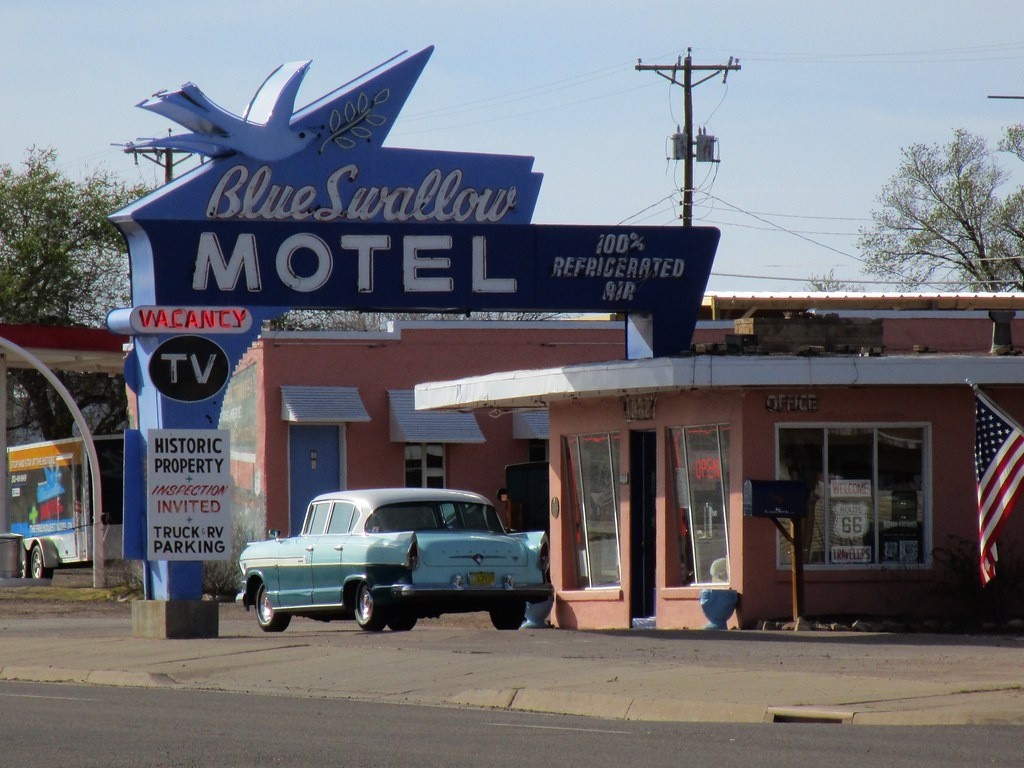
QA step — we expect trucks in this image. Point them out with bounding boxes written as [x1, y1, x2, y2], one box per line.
[6, 434, 124, 579]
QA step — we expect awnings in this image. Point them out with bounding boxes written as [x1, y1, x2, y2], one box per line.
[512, 406, 551, 440]
[388, 391, 488, 445]
[281, 384, 374, 425]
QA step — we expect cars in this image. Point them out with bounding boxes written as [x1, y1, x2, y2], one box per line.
[235, 488, 551, 632]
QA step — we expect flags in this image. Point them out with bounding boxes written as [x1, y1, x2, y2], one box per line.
[975, 392, 1024, 586]
